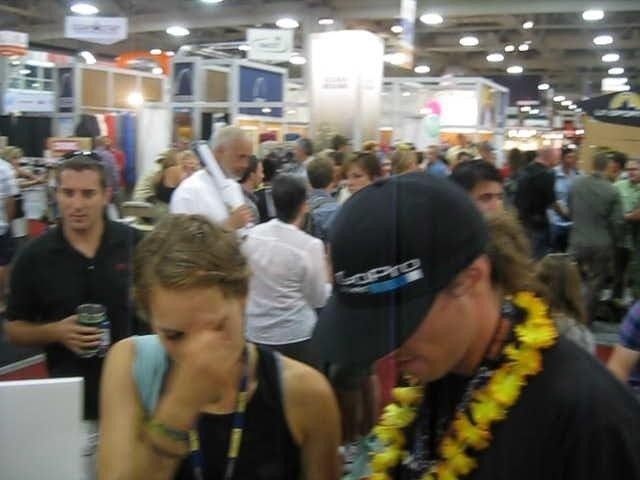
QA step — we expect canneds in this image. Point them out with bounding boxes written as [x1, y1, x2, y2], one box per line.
[76, 303, 113, 358]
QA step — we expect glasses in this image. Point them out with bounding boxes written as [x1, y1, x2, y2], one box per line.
[61, 150, 102, 162]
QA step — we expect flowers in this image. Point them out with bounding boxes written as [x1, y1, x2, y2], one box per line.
[361, 289, 561, 480]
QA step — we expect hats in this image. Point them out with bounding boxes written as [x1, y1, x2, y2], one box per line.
[304, 170, 490, 368]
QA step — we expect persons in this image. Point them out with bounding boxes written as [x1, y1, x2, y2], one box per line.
[1, 120, 640, 480]
